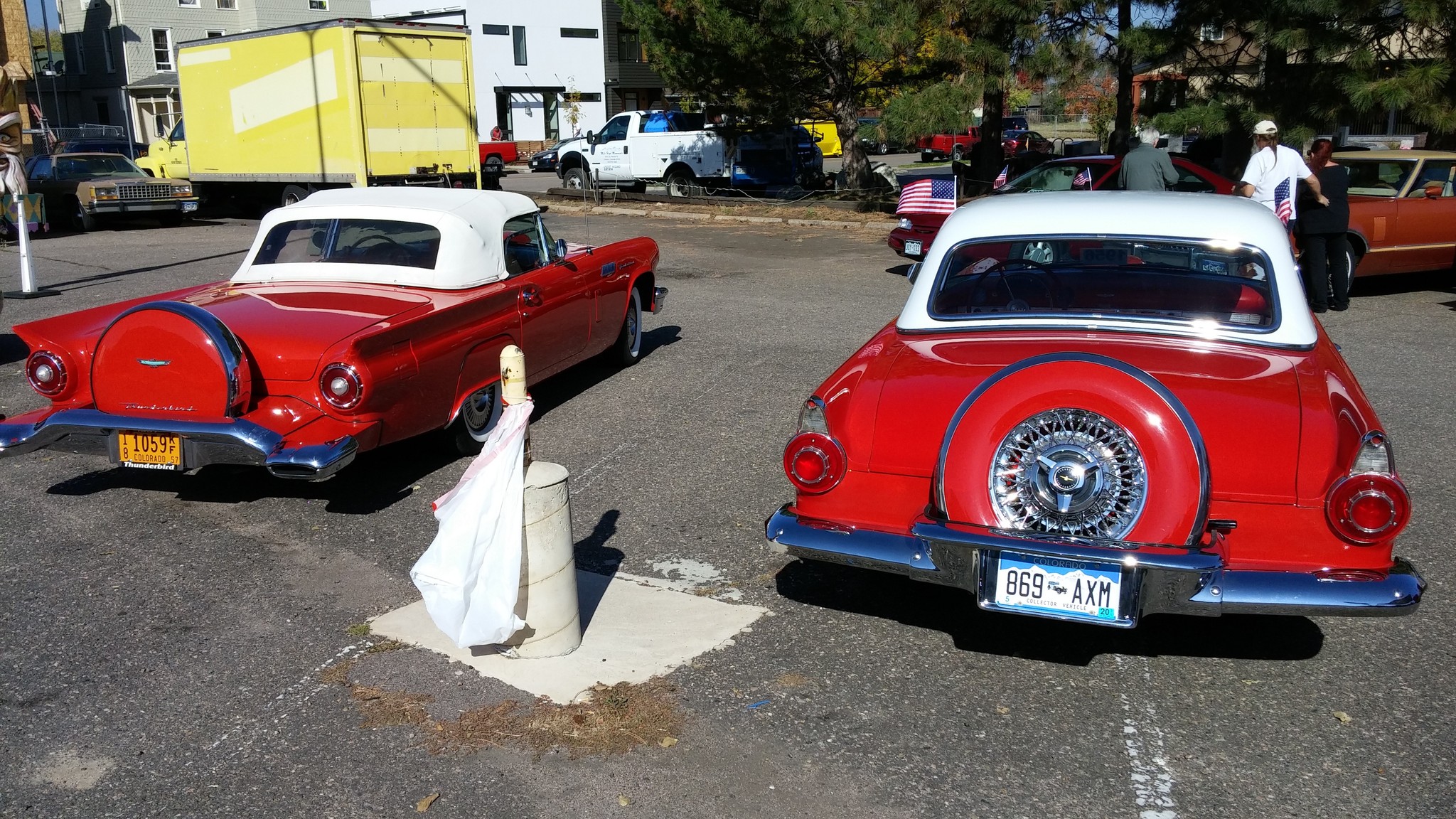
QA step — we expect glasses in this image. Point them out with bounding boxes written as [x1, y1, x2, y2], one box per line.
[1307, 150, 1313, 156]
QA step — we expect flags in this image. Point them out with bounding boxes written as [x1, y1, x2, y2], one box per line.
[993, 167, 1007, 189]
[1073, 170, 1092, 186]
[1274, 177, 1293, 227]
[896, 179, 955, 214]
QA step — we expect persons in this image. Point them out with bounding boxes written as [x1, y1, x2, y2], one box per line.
[1293, 138, 1350, 314]
[490, 126, 503, 142]
[1231, 120, 1330, 236]
[1118, 128, 1179, 192]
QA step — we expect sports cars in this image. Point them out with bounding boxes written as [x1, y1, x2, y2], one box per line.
[1, 187, 669, 483]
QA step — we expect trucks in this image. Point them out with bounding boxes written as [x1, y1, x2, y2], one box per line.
[133, 18, 483, 231]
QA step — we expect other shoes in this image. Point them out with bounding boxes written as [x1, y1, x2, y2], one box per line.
[1334, 303, 1348, 311]
[1315, 303, 1327, 313]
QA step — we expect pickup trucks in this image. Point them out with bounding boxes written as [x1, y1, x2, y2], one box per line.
[477, 142, 520, 172]
[915, 125, 983, 163]
[554, 109, 787, 197]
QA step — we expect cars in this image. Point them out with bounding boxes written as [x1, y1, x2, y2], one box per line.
[763, 191, 1430, 631]
[1286, 149, 1456, 299]
[527, 136, 588, 171]
[23, 152, 199, 232]
[784, 125, 824, 175]
[52, 137, 150, 162]
[886, 154, 1238, 262]
[1002, 129, 1055, 159]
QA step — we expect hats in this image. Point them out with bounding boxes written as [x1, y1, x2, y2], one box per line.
[1248, 120, 1277, 139]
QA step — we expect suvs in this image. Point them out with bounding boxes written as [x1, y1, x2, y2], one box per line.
[857, 114, 919, 154]
[1002, 117, 1028, 132]
